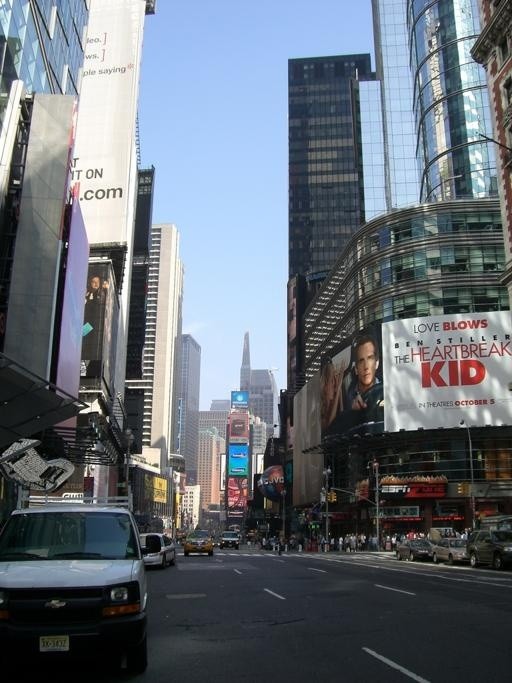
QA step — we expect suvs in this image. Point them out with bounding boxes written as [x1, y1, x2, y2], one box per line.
[1, 488, 153, 678]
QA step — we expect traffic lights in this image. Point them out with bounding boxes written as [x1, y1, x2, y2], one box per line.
[332, 492, 337, 502]
[327, 492, 332, 502]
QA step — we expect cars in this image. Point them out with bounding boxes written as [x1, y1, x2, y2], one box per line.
[267, 536, 286, 552]
[394, 526, 512, 568]
[139, 529, 240, 569]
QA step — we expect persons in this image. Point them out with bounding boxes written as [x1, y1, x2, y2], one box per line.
[100, 277, 110, 291]
[320, 357, 347, 432]
[246, 527, 473, 554]
[346, 332, 386, 410]
[84, 272, 106, 306]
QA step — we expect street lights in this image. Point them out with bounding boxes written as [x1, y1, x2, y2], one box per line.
[124, 425, 134, 497]
[371, 460, 382, 551]
[281, 487, 287, 546]
[322, 465, 331, 552]
[460, 417, 477, 535]
